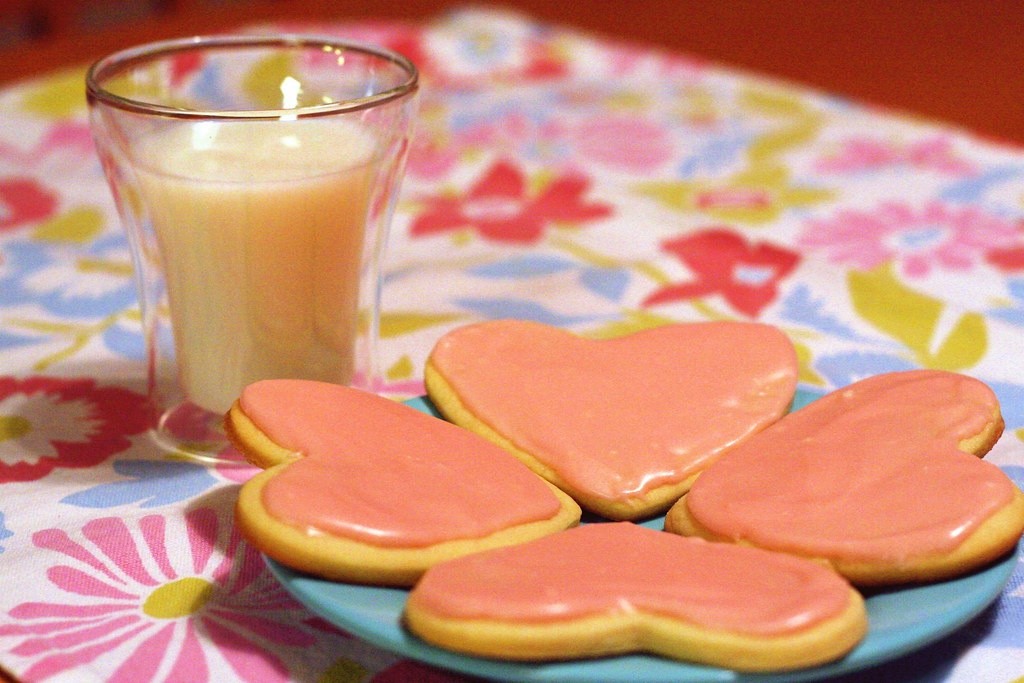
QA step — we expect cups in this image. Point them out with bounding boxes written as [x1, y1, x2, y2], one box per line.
[81, 38, 420, 465]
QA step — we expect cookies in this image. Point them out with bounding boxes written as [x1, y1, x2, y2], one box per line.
[223, 316, 1024, 673]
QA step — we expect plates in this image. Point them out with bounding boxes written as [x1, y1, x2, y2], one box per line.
[259, 392, 1020, 683]
